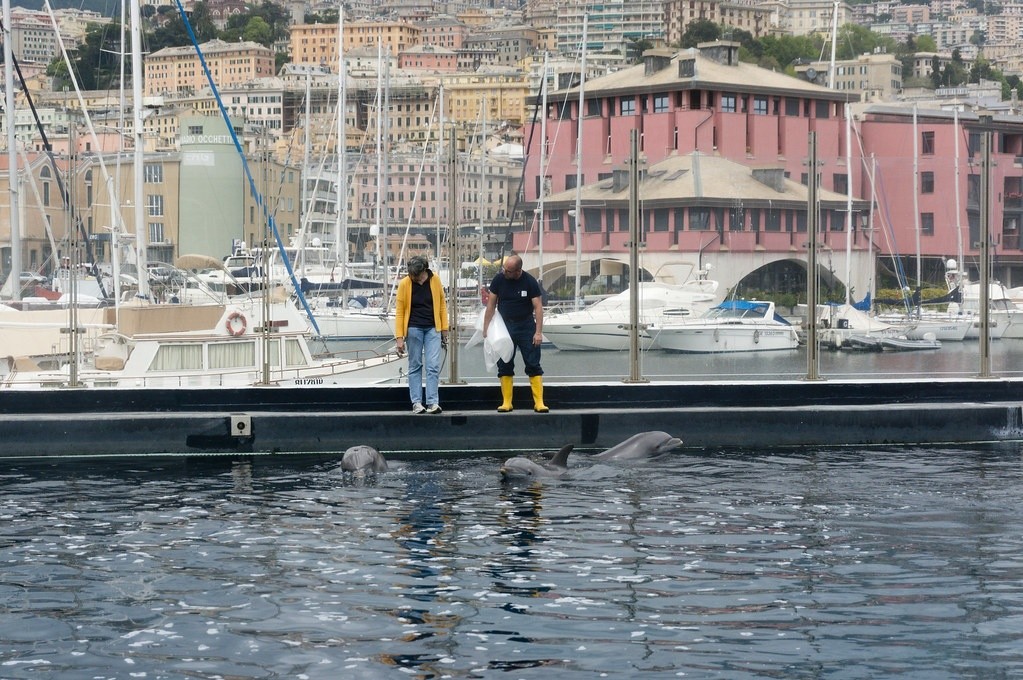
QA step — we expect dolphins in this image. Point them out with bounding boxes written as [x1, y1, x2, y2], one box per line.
[341, 445, 389, 475]
[500, 430, 685, 478]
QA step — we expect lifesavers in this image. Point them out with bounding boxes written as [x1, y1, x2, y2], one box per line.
[226, 313, 247, 337]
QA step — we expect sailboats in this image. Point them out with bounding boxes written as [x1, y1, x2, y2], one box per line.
[0, 0, 551, 390]
[765, 0, 1023, 353]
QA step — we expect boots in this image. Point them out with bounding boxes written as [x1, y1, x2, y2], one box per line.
[498, 375, 513, 412]
[529, 375, 549, 412]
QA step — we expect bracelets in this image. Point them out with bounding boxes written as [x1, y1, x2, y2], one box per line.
[536, 333, 541, 335]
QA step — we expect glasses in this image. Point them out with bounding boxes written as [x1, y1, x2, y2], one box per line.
[502, 265, 518, 274]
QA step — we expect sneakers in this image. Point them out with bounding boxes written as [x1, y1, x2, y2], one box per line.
[413, 402, 426, 414]
[427, 404, 443, 413]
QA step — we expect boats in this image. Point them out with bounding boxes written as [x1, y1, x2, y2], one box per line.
[643, 298, 800, 353]
[540, 262, 720, 352]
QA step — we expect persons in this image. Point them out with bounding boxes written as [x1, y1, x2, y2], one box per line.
[482, 255, 551, 413]
[396, 257, 449, 413]
